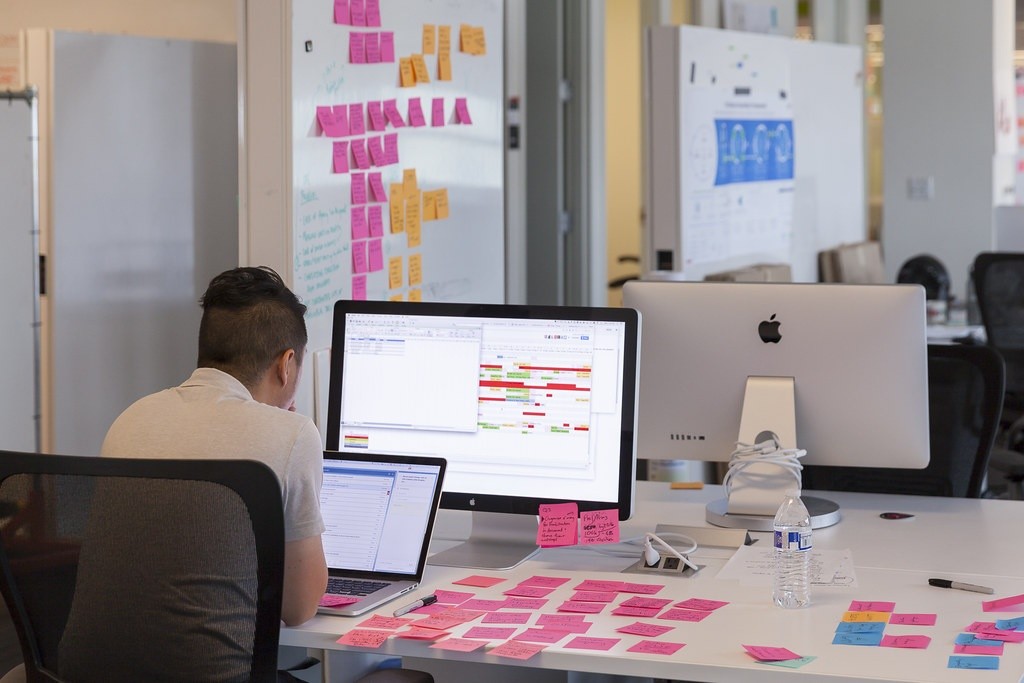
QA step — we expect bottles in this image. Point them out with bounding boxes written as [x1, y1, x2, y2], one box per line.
[770, 488, 813, 611]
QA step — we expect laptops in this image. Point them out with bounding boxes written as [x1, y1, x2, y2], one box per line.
[318, 451, 448, 617]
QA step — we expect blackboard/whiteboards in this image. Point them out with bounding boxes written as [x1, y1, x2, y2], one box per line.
[293, 0, 507, 353]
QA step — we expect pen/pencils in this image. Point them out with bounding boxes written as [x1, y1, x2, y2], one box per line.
[928, 579, 993, 594]
[392, 595, 438, 617]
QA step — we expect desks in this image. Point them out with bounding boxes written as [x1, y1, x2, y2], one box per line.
[278, 478, 1024, 683]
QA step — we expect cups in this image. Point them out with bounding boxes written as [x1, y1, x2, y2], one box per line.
[926, 299, 946, 324]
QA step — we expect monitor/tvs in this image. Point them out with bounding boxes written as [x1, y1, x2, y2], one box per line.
[621, 279, 930, 530]
[327, 297, 646, 572]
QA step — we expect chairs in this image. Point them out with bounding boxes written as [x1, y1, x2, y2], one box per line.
[0, 450, 435, 683]
[800, 344, 1006, 499]
[896, 255, 950, 302]
[952, 252, 1024, 397]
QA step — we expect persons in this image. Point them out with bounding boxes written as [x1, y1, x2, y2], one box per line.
[55, 267, 328, 683]
